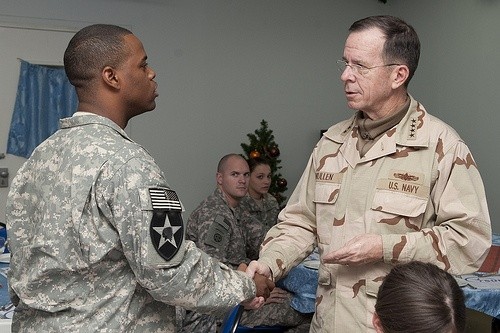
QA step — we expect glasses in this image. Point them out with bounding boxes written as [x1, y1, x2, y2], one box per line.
[336, 60, 400, 75]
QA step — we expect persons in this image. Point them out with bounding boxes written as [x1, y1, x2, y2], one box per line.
[243, 16, 491, 333]
[372, 260, 466, 333]
[185, 154, 316, 333]
[6, 25, 275, 333]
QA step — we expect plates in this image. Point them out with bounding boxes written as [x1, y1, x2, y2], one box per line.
[303, 260, 320, 270]
[0, 253, 11, 263]
[455, 278, 468, 287]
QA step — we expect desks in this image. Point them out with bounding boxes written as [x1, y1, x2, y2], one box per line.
[274, 231, 500, 333]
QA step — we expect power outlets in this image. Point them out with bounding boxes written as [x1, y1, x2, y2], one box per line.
[0, 167, 8, 187]
